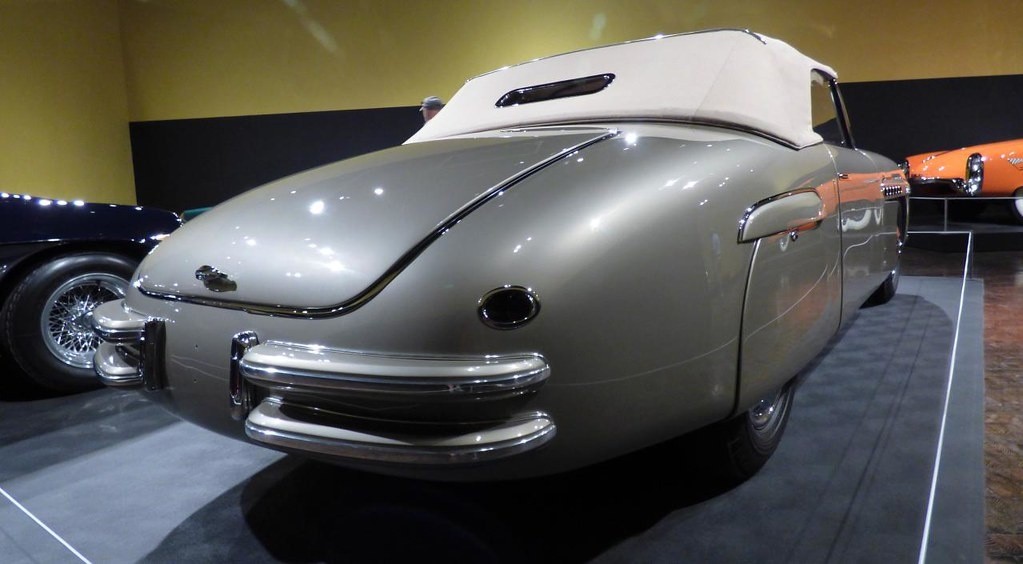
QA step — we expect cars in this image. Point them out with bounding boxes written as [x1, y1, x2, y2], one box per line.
[0, 194, 185, 394]
[897, 138, 1023, 223]
[94, 28, 912, 486]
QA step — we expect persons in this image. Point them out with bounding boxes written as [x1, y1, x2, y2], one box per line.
[419, 96, 443, 122]
